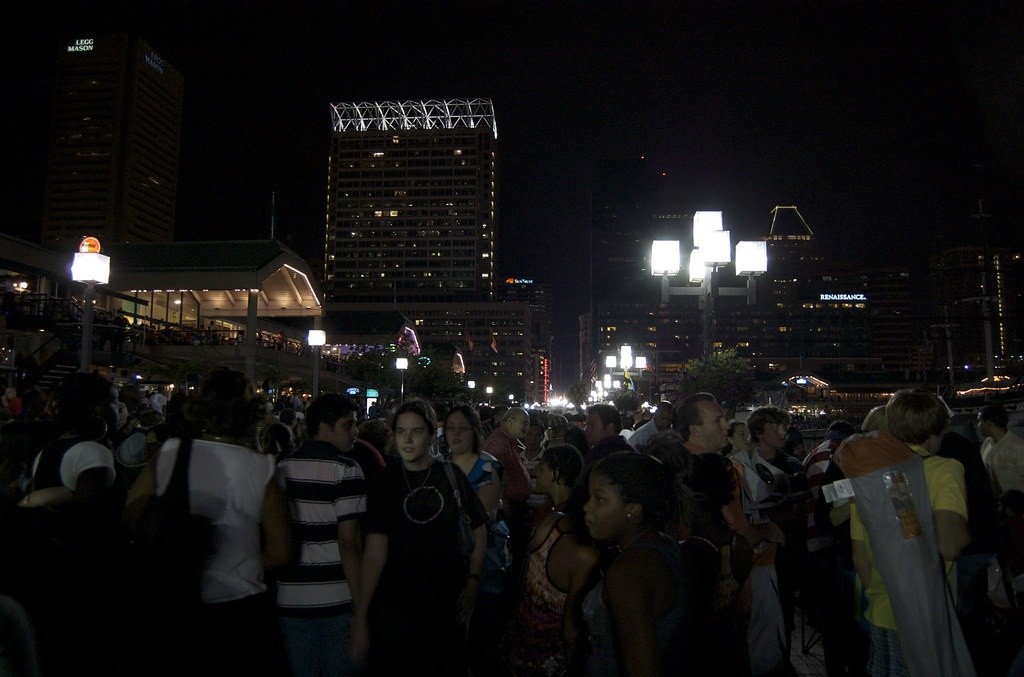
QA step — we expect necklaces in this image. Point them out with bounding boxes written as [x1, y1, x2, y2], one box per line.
[397, 460, 433, 496]
[206, 434, 240, 445]
[618, 523, 652, 552]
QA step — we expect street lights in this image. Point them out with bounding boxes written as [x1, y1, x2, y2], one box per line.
[650, 211, 767, 357]
[486, 387, 492, 405]
[308, 330, 326, 400]
[509, 394, 514, 404]
[71, 252, 111, 371]
[396, 358, 408, 406]
[606, 346, 646, 417]
[468, 381, 475, 407]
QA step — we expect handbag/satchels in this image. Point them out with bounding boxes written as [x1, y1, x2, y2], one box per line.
[134, 435, 220, 578]
[444, 462, 489, 631]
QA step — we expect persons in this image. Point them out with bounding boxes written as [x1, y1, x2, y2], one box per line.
[0, 311, 1024, 677]
